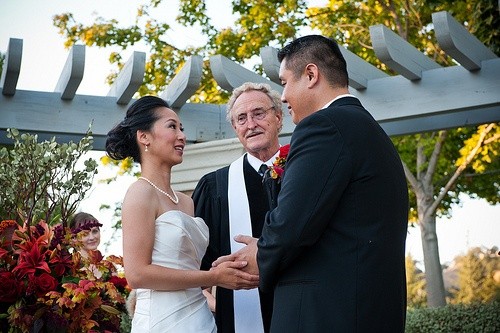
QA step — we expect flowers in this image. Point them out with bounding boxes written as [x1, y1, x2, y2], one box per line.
[268, 144, 292, 183]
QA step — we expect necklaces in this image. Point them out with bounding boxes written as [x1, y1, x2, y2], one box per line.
[139, 176, 179, 206]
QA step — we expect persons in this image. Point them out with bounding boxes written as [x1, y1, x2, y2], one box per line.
[68, 212, 105, 281]
[211, 34, 409, 333]
[105, 95, 259, 333]
[192, 82, 289, 333]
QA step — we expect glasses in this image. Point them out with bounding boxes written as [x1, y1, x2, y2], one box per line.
[235, 106, 276, 124]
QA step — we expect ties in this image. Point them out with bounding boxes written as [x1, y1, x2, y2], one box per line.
[258, 165, 277, 210]
